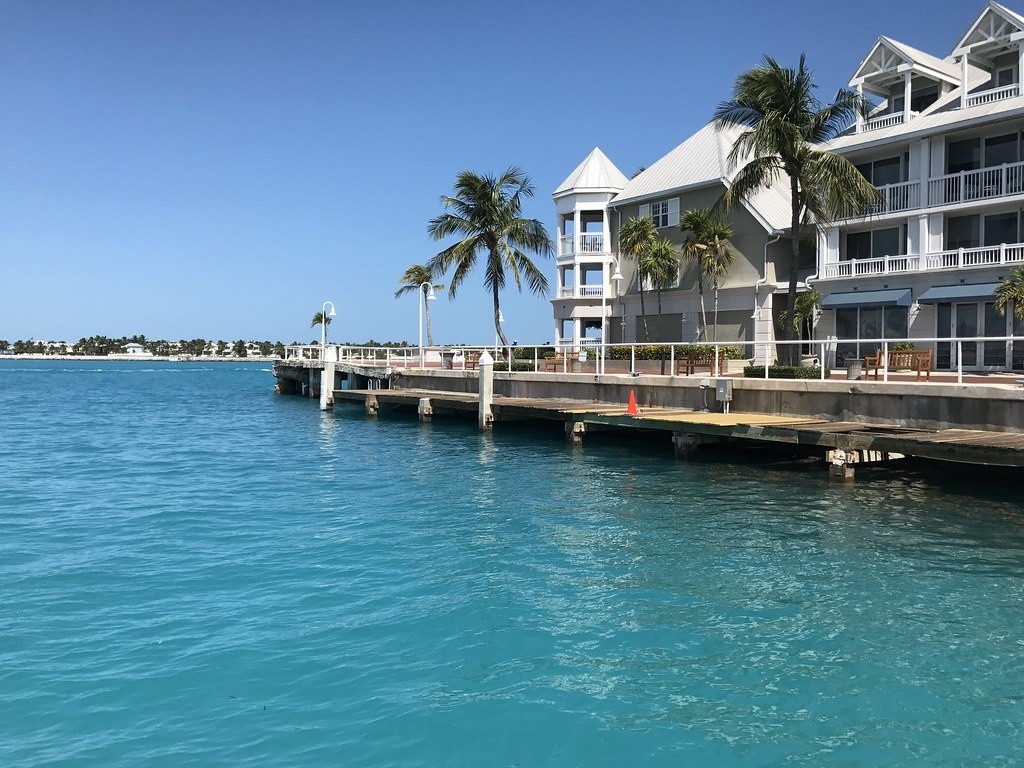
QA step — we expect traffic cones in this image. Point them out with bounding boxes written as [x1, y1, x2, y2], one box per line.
[624, 390, 640, 415]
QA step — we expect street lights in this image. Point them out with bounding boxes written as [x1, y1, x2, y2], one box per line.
[750, 305, 770, 379]
[419, 282, 437, 367]
[600, 256, 623, 374]
[495, 310, 504, 362]
[322, 302, 337, 360]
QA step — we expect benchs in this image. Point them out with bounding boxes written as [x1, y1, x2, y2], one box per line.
[465, 352, 494, 370]
[865, 348, 932, 382]
[677, 351, 724, 376]
[543, 351, 579, 372]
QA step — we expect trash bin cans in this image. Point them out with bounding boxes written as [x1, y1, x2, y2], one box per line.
[845, 359, 865, 379]
[439, 352, 455, 370]
